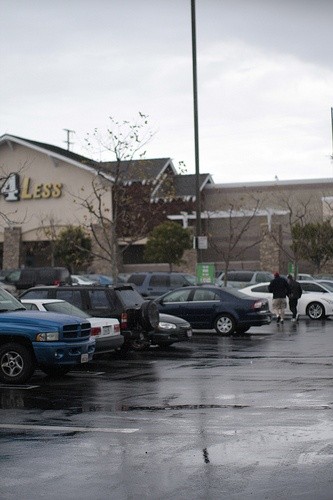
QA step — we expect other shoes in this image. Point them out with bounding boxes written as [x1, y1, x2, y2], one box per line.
[291, 319, 296, 321]
[276, 315, 281, 323]
[280, 319, 284, 324]
[296, 313, 300, 321]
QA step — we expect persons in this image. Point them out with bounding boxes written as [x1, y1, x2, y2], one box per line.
[287, 275, 302, 322]
[269, 272, 289, 324]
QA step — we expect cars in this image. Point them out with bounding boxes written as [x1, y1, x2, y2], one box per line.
[0, 299, 124, 356]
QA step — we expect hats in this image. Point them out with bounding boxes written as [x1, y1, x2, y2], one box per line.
[274, 273, 279, 278]
[287, 275, 293, 280]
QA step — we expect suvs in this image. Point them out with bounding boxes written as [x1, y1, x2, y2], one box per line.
[0, 288, 93, 386]
[0, 268, 333, 352]
